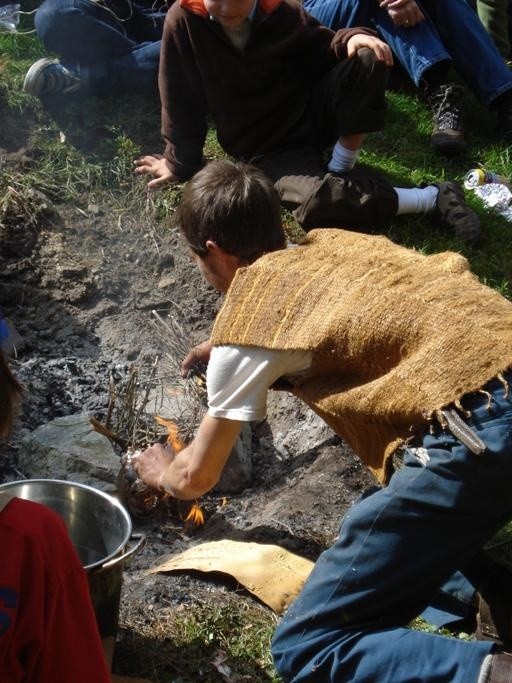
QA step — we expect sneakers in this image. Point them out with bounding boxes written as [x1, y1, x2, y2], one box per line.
[22, 59, 80, 94]
[429, 180, 481, 242]
[430, 89, 468, 152]
[322, 163, 377, 190]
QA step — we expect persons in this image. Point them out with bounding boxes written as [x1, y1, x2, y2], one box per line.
[305, 1, 511, 156]
[1, 315, 115, 676]
[133, 1, 483, 250]
[129, 158, 512, 682]
[20, 0, 181, 97]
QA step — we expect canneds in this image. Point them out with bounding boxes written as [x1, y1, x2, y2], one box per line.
[465, 169, 511, 191]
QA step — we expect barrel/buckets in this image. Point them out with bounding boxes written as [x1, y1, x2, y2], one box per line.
[0, 479, 147, 676]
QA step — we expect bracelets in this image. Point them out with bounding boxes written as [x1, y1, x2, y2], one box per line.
[154, 468, 169, 496]
